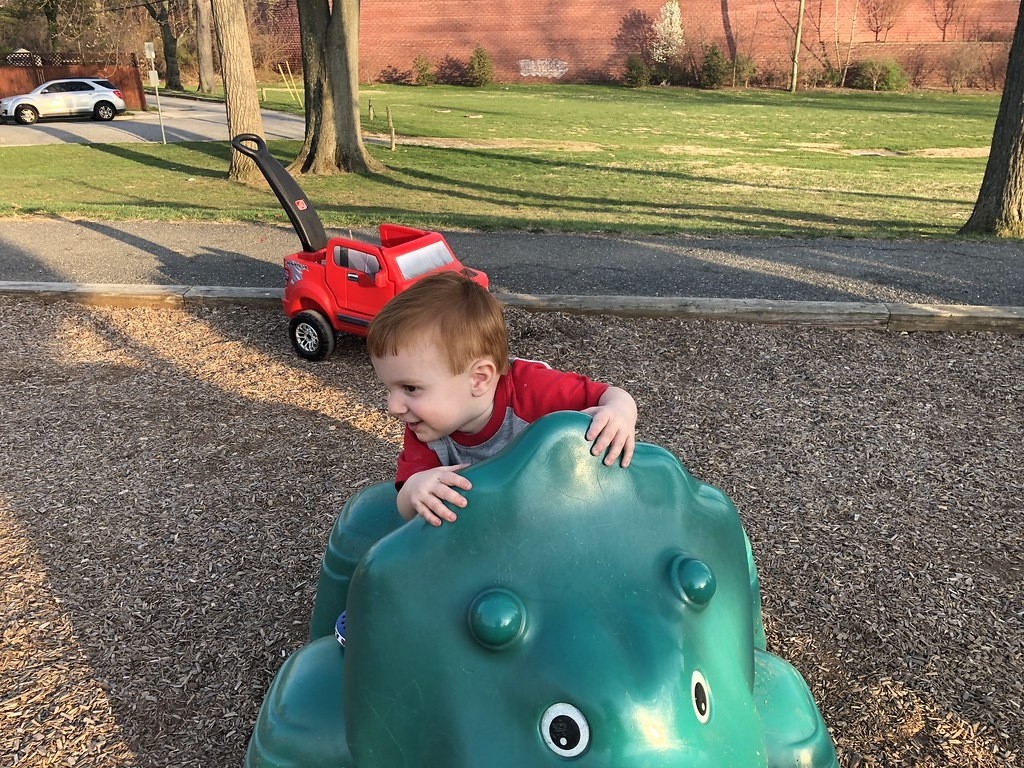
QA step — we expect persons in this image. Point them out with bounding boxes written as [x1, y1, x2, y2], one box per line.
[366, 271, 638, 526]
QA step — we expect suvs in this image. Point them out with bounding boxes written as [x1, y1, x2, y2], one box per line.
[0, 77, 127, 126]
